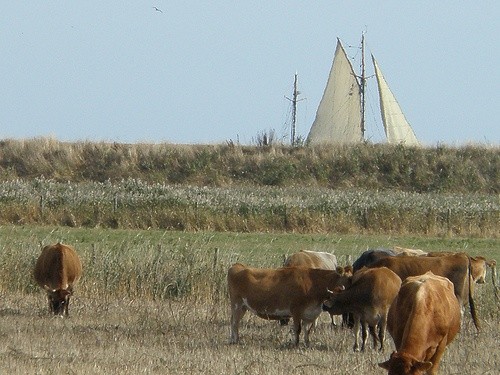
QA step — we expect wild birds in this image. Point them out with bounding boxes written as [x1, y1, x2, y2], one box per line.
[489, 258, 500, 317]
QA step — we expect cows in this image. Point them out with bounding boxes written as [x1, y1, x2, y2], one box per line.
[226, 262, 403, 354]
[342, 246, 494, 329]
[378, 270, 462, 374]
[285, 250, 338, 326]
[34, 241, 82, 318]
